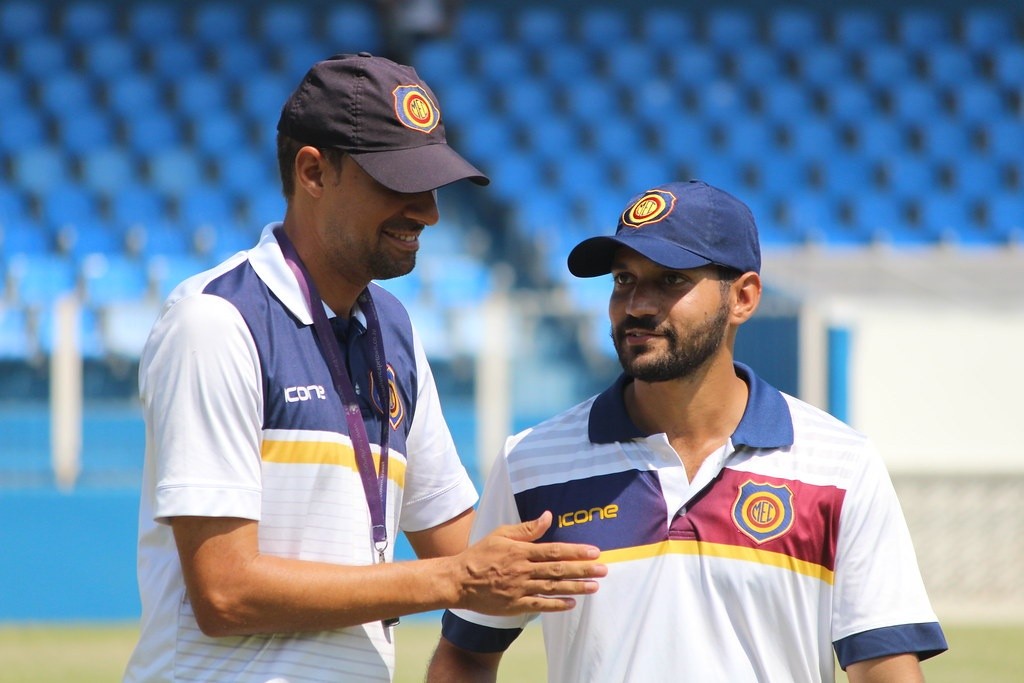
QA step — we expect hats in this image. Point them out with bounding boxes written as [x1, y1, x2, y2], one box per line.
[276, 50, 492, 194]
[568, 178, 763, 280]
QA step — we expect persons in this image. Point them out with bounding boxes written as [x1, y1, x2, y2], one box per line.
[425, 179, 949, 683]
[120, 51, 608, 683]
